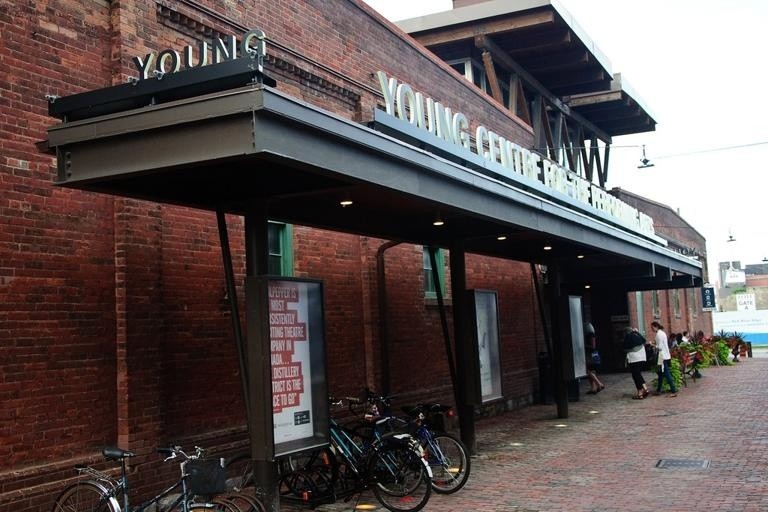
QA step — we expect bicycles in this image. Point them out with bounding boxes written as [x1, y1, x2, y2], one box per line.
[51, 443, 224, 512]
[208, 390, 471, 512]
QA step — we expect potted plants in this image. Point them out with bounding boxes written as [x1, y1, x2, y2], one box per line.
[680, 329, 711, 378]
[727, 331, 745, 362]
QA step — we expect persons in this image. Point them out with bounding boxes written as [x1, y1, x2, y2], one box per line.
[619, 325, 650, 399]
[646, 330, 706, 367]
[582, 321, 605, 396]
[648, 321, 679, 397]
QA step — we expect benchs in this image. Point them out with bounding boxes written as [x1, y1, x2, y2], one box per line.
[680, 351, 697, 388]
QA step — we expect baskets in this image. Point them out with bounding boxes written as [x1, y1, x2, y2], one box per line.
[190, 458, 226, 495]
